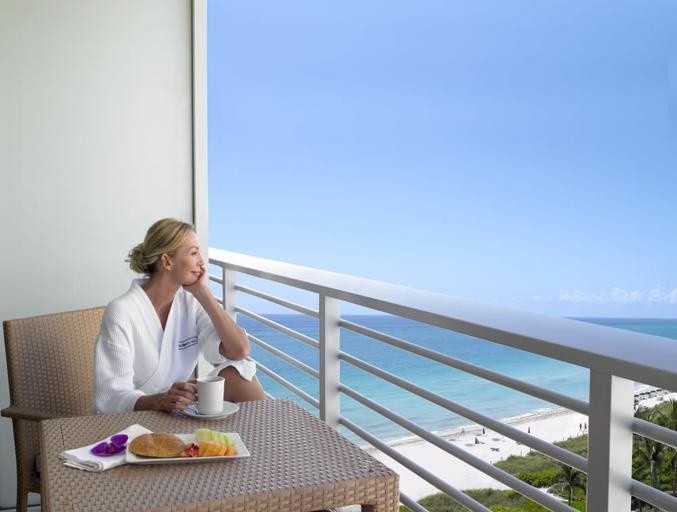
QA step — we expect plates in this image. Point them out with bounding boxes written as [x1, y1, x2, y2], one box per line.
[183, 402, 239, 420]
[124, 432, 253, 466]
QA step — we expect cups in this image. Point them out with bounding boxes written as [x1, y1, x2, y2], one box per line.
[191, 376, 226, 416]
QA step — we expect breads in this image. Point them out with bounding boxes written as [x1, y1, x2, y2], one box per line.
[129, 433, 186, 457]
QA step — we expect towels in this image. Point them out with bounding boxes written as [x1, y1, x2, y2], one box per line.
[60, 422, 152, 474]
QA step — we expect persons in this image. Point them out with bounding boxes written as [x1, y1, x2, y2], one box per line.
[92, 218, 267, 417]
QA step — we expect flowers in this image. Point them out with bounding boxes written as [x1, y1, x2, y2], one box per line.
[90, 432, 128, 457]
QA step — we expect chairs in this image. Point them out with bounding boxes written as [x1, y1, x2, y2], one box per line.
[0, 306, 108, 511]
